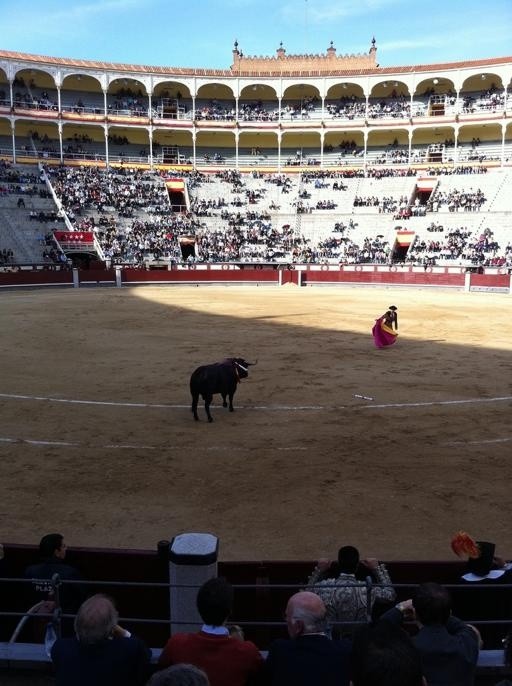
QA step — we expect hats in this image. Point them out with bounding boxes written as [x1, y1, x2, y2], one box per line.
[388, 306, 397, 309]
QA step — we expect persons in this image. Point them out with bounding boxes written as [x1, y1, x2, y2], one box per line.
[267, 591, 353, 686]
[381, 582, 483, 686]
[348, 618, 428, 686]
[1, 76, 512, 280]
[50, 595, 153, 686]
[304, 544, 395, 641]
[375, 305, 398, 334]
[17, 533, 86, 642]
[155, 576, 264, 686]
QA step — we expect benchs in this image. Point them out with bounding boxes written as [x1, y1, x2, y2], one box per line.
[1, 84, 512, 275]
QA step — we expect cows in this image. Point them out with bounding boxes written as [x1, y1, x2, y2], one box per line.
[189, 356, 259, 424]
